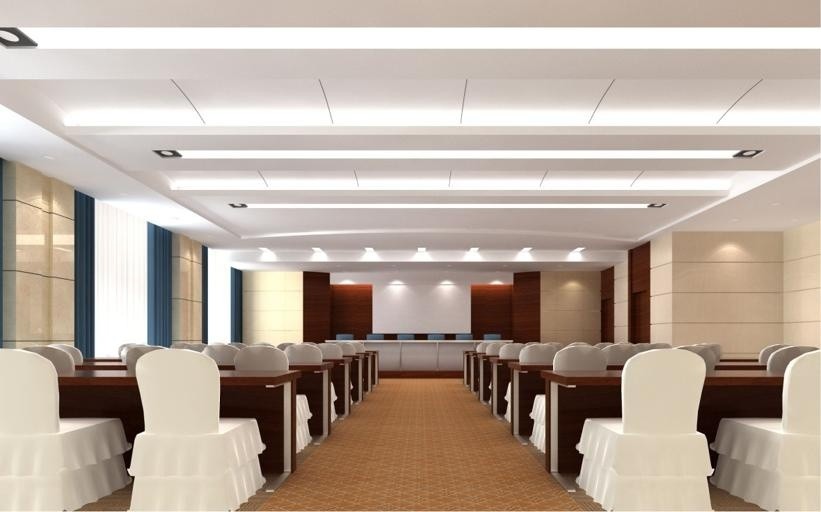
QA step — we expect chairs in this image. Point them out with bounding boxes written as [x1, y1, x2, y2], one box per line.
[334, 333, 502, 341]
[476, 340, 816, 453]
[0, 350, 120, 508]
[20, 343, 367, 454]
[722, 347, 821, 510]
[136, 349, 258, 510]
[587, 347, 709, 510]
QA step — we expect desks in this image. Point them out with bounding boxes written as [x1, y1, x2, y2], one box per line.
[323, 339, 513, 378]
[476, 358, 783, 492]
[51, 358, 379, 491]
